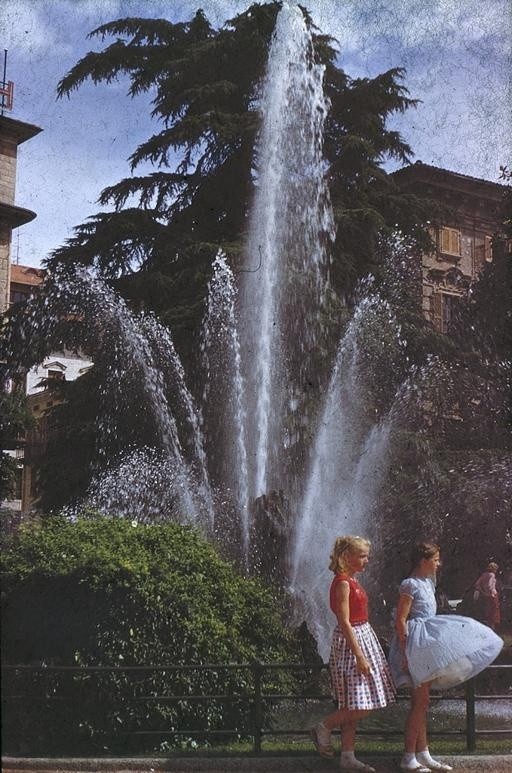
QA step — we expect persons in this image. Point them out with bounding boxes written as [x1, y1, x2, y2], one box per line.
[306, 534, 398, 773]
[476, 561, 501, 632]
[389, 541, 505, 773]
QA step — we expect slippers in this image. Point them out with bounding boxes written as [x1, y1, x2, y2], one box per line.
[429, 762, 447, 773]
[339, 763, 376, 773]
[309, 728, 336, 760]
[400, 765, 431, 772]
